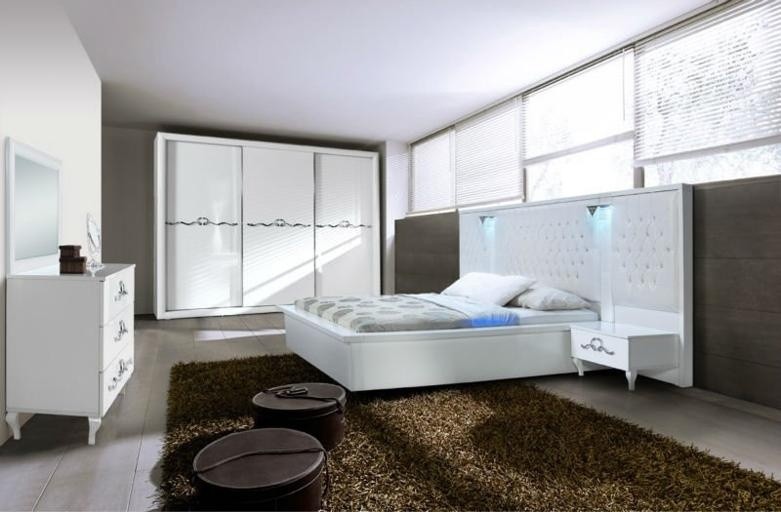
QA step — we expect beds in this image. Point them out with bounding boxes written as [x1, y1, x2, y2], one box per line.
[277, 292, 599, 393]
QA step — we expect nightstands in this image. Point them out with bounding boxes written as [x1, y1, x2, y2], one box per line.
[570, 321, 680, 391]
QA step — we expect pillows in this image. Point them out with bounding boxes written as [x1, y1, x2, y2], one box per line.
[440, 271, 537, 307]
[509, 285, 591, 311]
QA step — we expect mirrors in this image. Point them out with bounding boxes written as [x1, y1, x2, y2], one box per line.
[5, 136, 63, 275]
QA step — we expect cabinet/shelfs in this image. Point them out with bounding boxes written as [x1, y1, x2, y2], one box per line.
[5, 262, 137, 446]
[152, 130, 381, 320]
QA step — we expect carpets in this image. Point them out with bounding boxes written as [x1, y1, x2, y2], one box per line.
[149, 351, 781, 512]
